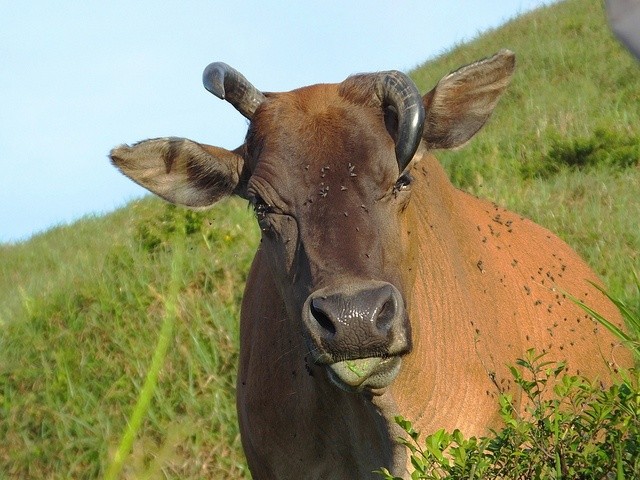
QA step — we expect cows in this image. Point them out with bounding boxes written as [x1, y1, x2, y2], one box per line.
[109, 50, 634, 479]
